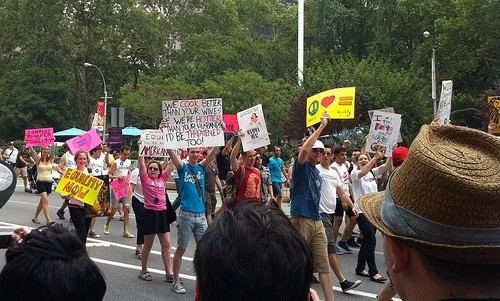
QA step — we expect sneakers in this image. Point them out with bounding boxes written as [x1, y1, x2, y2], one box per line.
[56, 210, 65, 220]
[347, 236, 362, 247]
[104, 224, 110, 235]
[339, 279, 361, 293]
[67, 223, 75, 232]
[122, 231, 134, 238]
[88, 232, 101, 238]
[171, 282, 187, 294]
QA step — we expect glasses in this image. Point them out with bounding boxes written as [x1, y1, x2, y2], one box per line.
[148, 167, 159, 171]
[310, 149, 324, 153]
[255, 156, 262, 161]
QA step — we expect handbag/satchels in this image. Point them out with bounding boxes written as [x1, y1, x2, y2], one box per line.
[166, 194, 177, 225]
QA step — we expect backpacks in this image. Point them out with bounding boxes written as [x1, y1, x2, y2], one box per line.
[224, 165, 245, 207]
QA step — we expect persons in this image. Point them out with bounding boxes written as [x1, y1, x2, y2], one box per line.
[0, 111, 440, 301]
[357, 123, 500, 301]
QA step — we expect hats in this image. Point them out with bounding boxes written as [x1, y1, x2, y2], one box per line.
[8, 141, 14, 145]
[358, 124, 500, 248]
[311, 140, 325, 149]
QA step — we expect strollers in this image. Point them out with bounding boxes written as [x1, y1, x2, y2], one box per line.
[27, 162, 38, 194]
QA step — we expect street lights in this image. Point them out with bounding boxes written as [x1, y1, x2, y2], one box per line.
[423, 29, 437, 117]
[84, 62, 107, 145]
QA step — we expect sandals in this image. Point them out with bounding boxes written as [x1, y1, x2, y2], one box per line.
[137, 270, 153, 281]
[165, 274, 183, 284]
[119, 217, 124, 221]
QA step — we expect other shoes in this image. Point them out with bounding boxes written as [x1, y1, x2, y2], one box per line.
[135, 250, 150, 260]
[338, 241, 352, 253]
[31, 217, 40, 224]
[370, 274, 386, 282]
[46, 221, 55, 225]
[334, 246, 343, 254]
[356, 270, 369, 277]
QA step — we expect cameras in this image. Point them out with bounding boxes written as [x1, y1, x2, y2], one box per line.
[0, 232, 26, 250]
